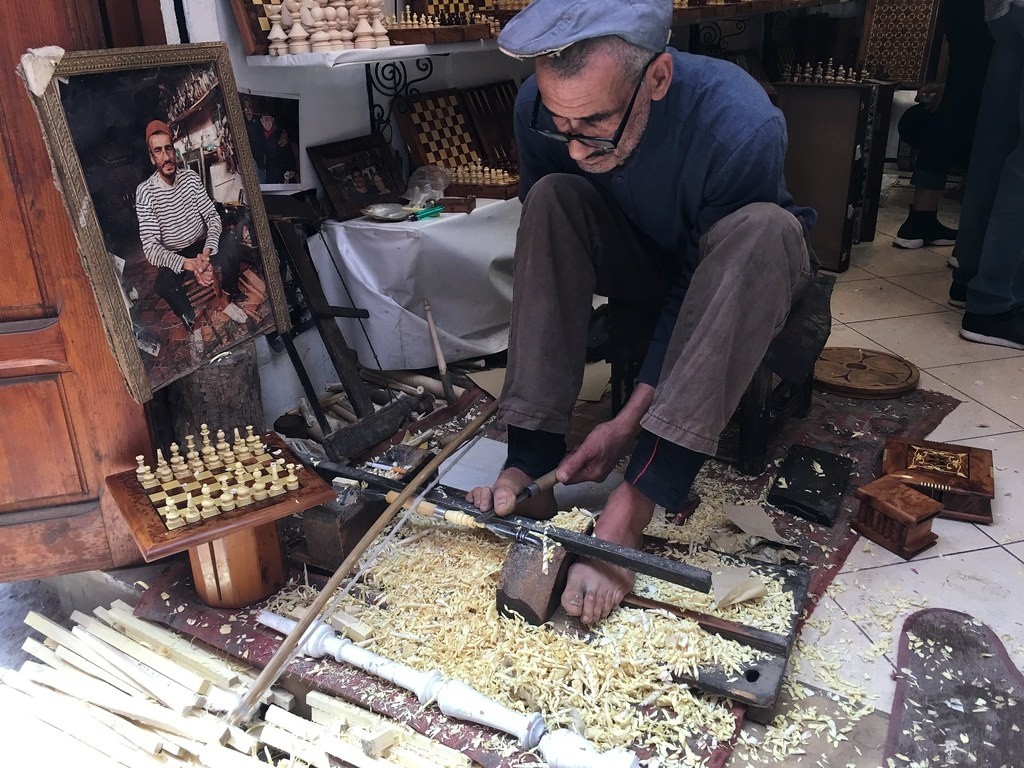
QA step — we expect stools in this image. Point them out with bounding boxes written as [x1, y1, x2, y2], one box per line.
[609, 272, 837, 456]
[182, 268, 221, 307]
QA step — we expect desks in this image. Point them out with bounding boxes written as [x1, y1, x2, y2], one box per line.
[306, 194, 523, 371]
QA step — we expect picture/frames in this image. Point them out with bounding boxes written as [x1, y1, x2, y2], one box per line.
[182, 147, 207, 189]
[15, 40, 291, 404]
[306, 132, 407, 222]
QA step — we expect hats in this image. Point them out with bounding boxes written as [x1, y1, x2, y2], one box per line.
[498, 0, 674, 58]
[146, 120, 172, 146]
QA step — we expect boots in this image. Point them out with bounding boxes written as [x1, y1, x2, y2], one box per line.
[892, 203, 958, 248]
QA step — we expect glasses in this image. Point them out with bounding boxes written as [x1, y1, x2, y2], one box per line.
[527, 53, 655, 152]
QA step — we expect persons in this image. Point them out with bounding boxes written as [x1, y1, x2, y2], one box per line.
[891, 0, 1024, 250]
[134, 95, 395, 334]
[460, 0, 838, 628]
[948, 0, 1024, 349]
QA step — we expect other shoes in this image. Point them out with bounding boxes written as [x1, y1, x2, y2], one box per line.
[219, 301, 246, 323]
[186, 325, 205, 363]
[960, 309, 1023, 349]
[949, 278, 973, 307]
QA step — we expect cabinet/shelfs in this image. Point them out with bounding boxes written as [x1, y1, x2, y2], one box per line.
[167, 81, 219, 164]
